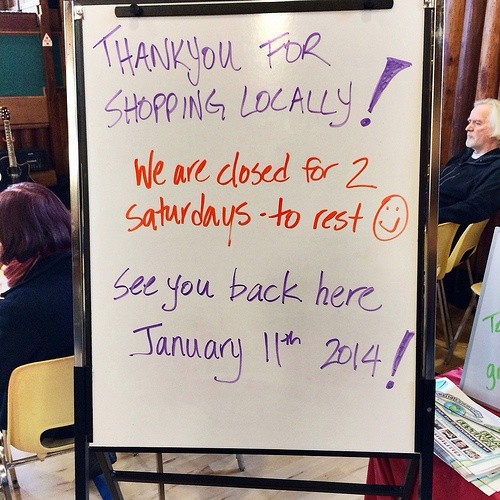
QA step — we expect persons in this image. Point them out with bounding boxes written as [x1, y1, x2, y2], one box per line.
[434, 99, 500, 313]
[0, 182, 74, 486]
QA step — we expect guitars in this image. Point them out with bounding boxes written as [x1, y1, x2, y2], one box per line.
[0, 106, 33, 190]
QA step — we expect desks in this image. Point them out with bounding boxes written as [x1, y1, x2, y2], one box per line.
[364, 366, 500, 500]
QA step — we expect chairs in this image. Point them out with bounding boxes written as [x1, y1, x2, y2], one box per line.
[0, 353, 74, 500]
[437, 217, 489, 366]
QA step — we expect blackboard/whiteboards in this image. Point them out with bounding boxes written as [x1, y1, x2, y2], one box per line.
[58, 0, 444, 456]
[461, 229, 500, 415]
